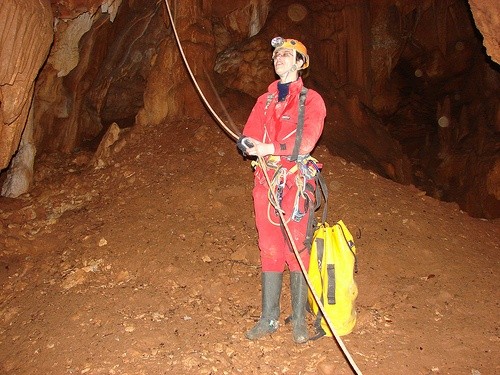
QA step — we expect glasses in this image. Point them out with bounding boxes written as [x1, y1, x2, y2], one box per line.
[274, 52, 294, 58]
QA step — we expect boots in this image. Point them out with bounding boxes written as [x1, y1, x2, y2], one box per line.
[289, 270, 311, 344]
[248, 272, 283, 339]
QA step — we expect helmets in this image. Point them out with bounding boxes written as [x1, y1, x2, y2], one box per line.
[272, 39, 310, 70]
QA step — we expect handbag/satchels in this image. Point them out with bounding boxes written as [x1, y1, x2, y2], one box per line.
[307, 220, 359, 337]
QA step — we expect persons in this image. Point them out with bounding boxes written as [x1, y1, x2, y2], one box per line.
[238, 37, 328, 343]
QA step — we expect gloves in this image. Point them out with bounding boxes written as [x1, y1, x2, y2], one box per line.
[238, 136, 255, 158]
